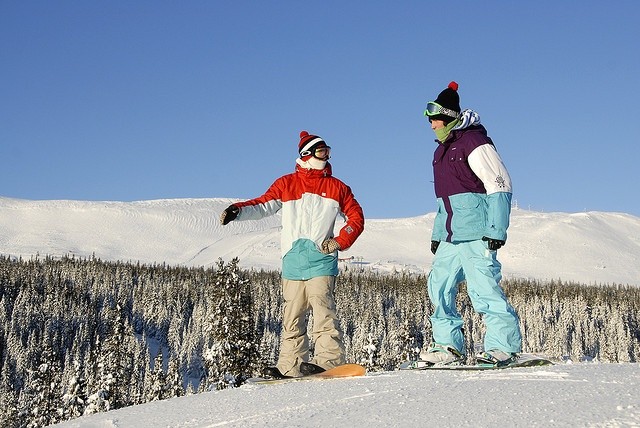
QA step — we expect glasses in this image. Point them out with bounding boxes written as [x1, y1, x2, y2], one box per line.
[424, 102, 459, 121]
[300, 146, 332, 161]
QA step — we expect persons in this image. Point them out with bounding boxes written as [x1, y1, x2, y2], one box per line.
[420, 80, 523, 365]
[219, 130, 365, 380]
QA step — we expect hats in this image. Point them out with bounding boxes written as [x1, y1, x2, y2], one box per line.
[428, 81, 460, 123]
[298, 130, 326, 157]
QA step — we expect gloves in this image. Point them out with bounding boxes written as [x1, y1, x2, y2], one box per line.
[321, 237, 340, 255]
[220, 204, 240, 225]
[480, 236, 505, 251]
[430, 240, 440, 255]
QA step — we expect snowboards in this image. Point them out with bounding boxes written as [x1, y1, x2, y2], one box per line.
[400, 354, 555, 369]
[246, 364, 366, 384]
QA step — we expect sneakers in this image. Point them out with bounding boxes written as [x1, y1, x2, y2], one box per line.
[263, 365, 294, 380]
[476, 348, 516, 365]
[417, 348, 464, 364]
[298, 360, 325, 376]
